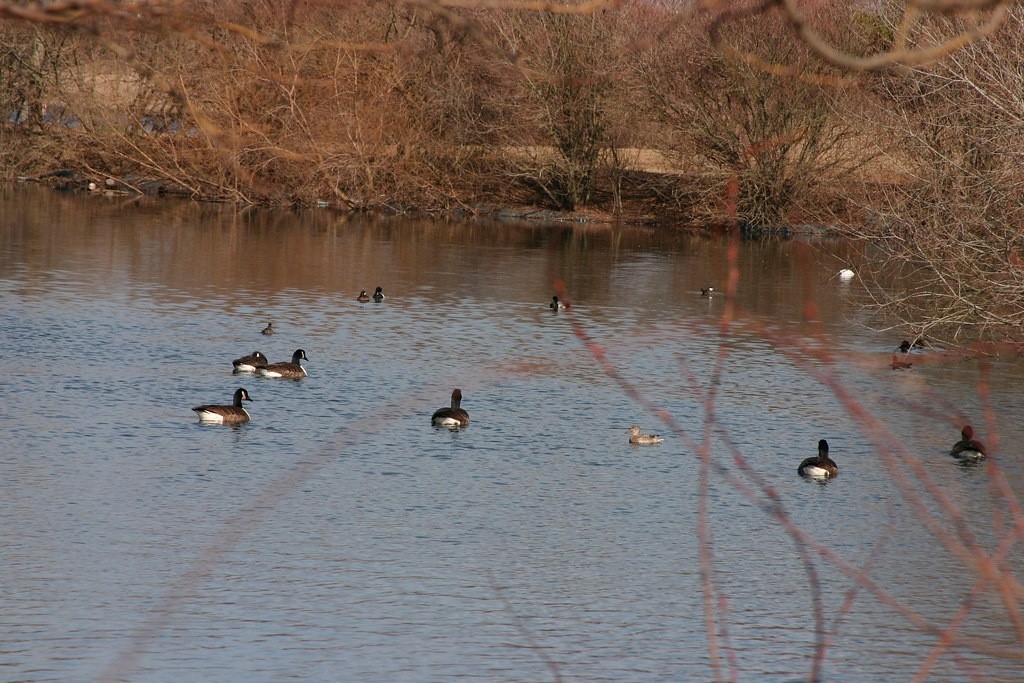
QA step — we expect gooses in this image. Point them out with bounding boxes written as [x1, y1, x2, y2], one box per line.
[799, 440, 837, 482]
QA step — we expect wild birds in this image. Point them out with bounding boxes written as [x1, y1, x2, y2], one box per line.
[836, 268, 855, 281]
[899, 340, 945, 355]
[890, 355, 913, 371]
[373, 287, 385, 303]
[951, 425, 984, 461]
[357, 290, 369, 301]
[625, 425, 664, 445]
[550, 296, 566, 312]
[263, 323, 273, 335]
[432, 389, 470, 427]
[232, 351, 268, 373]
[256, 349, 308, 379]
[191, 388, 253, 424]
[701, 286, 716, 300]
